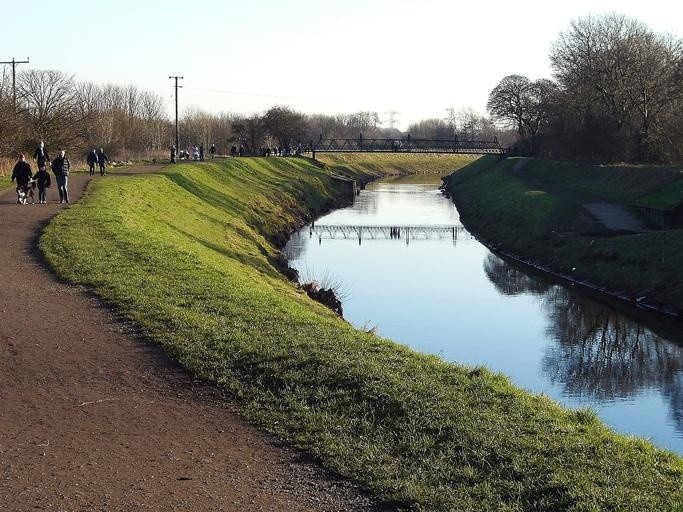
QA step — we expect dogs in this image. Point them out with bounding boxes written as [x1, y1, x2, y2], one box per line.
[16, 181, 36, 206]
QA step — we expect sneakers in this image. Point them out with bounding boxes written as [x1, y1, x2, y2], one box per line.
[60, 199, 69, 204]
[38, 200, 46, 204]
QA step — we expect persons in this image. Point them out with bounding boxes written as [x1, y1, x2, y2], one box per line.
[209, 144, 216, 159]
[12, 154, 32, 204]
[170, 144, 176, 163]
[33, 142, 51, 170]
[52, 150, 71, 203]
[179, 143, 205, 162]
[231, 144, 244, 158]
[87, 148, 98, 177]
[259, 147, 281, 157]
[32, 165, 51, 204]
[98, 148, 110, 177]
